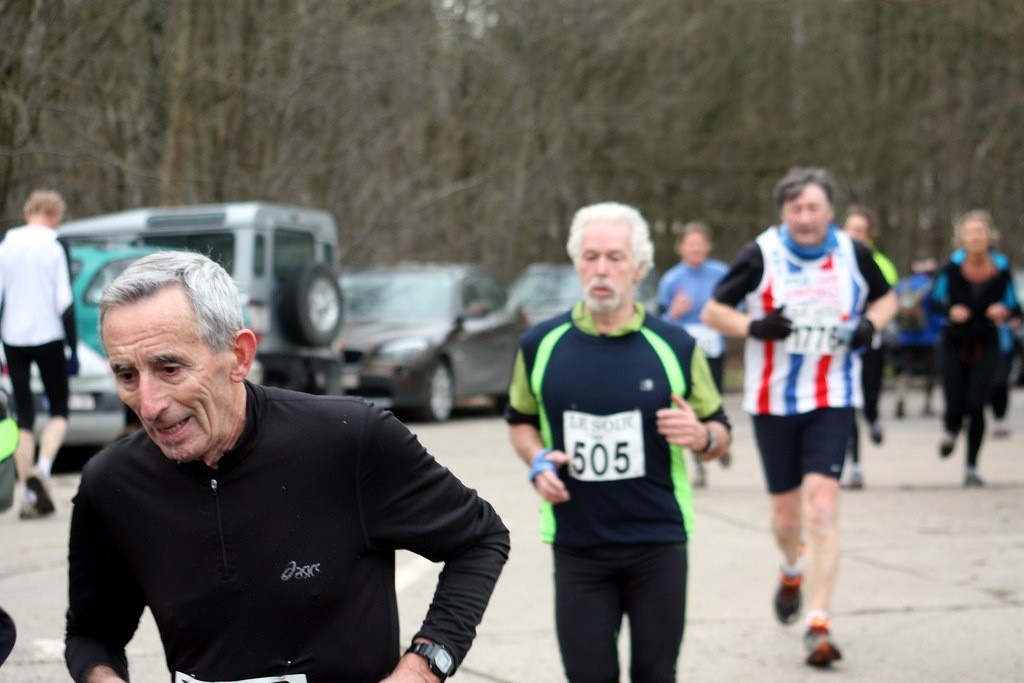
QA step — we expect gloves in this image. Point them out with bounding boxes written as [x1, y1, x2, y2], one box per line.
[850, 319, 875, 350]
[748, 305, 793, 341]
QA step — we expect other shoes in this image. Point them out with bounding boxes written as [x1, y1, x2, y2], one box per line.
[870, 421, 881, 443]
[965, 472, 982, 487]
[25, 467, 55, 515]
[19, 500, 38, 518]
[851, 468, 863, 488]
[721, 449, 729, 466]
[694, 472, 705, 488]
[941, 426, 957, 457]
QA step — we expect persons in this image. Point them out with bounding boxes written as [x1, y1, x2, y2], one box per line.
[505, 200, 732, 683]
[653, 165, 1024, 670]
[0, 189, 81, 518]
[63, 250, 511, 683]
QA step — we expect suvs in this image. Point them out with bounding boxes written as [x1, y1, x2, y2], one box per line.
[65, 238, 199, 360]
[53, 198, 346, 395]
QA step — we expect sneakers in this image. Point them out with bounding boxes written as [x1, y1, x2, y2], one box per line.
[774, 540, 805, 624]
[803, 615, 841, 665]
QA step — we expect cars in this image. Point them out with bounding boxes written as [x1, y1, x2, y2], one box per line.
[508, 260, 664, 326]
[0, 331, 129, 475]
[312, 258, 533, 424]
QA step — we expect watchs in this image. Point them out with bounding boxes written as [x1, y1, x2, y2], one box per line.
[403, 640, 455, 683]
[692, 424, 715, 456]
